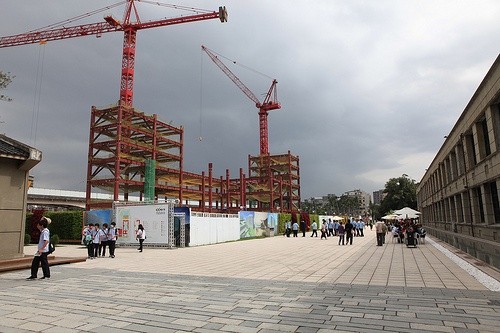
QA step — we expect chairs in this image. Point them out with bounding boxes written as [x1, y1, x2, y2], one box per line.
[392, 232, 427, 245]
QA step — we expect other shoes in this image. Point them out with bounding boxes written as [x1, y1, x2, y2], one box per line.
[94, 256, 97, 259]
[140, 251, 142, 252]
[40, 276, 50, 279]
[139, 248, 141, 250]
[99, 254, 101, 257]
[27, 276, 37, 279]
[112, 255, 115, 258]
[109, 255, 112, 257]
[90, 256, 93, 259]
[102, 255, 105, 258]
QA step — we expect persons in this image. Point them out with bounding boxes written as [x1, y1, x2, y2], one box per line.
[345, 219, 353, 245]
[283, 219, 292, 238]
[136, 224, 145, 252]
[82, 222, 118, 259]
[300, 219, 309, 237]
[376, 219, 388, 246]
[309, 220, 318, 237]
[351, 217, 364, 237]
[292, 220, 299, 238]
[26, 217, 50, 280]
[366, 221, 373, 231]
[385, 221, 424, 243]
[320, 219, 339, 240]
[338, 222, 344, 246]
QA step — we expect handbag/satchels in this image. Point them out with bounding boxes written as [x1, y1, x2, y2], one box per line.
[46, 229, 55, 255]
[351, 229, 354, 234]
[137, 235, 142, 238]
[86, 240, 93, 245]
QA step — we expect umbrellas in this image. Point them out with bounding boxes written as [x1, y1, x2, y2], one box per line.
[332, 216, 344, 221]
[380, 207, 422, 221]
